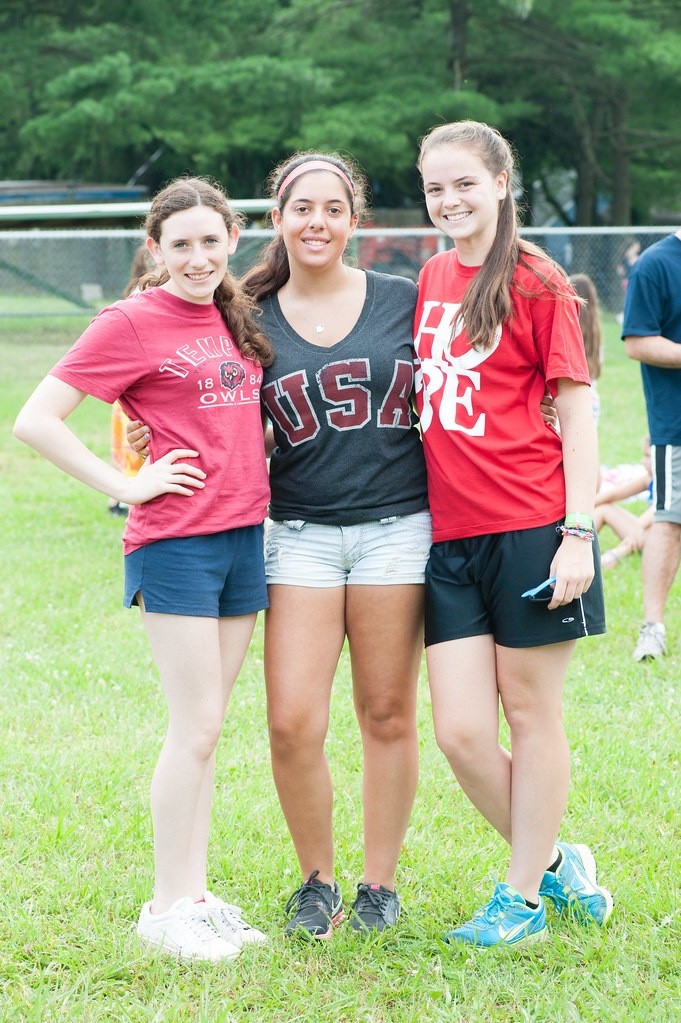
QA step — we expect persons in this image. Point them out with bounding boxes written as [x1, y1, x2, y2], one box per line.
[565, 273, 602, 431]
[416, 120, 610, 944]
[621, 226, 680, 662]
[10, 179, 277, 966]
[124, 151, 564, 942]
[592, 437, 655, 570]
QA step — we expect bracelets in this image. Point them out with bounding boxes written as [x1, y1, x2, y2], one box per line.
[558, 509, 595, 542]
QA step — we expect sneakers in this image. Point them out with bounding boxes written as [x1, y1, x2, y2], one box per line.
[184, 889, 266, 950]
[439, 882, 551, 952]
[344, 883, 402, 944]
[537, 840, 615, 932]
[135, 897, 244, 968]
[633, 618, 669, 663]
[280, 868, 347, 946]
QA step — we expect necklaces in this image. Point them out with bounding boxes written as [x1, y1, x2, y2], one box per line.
[285, 265, 350, 333]
